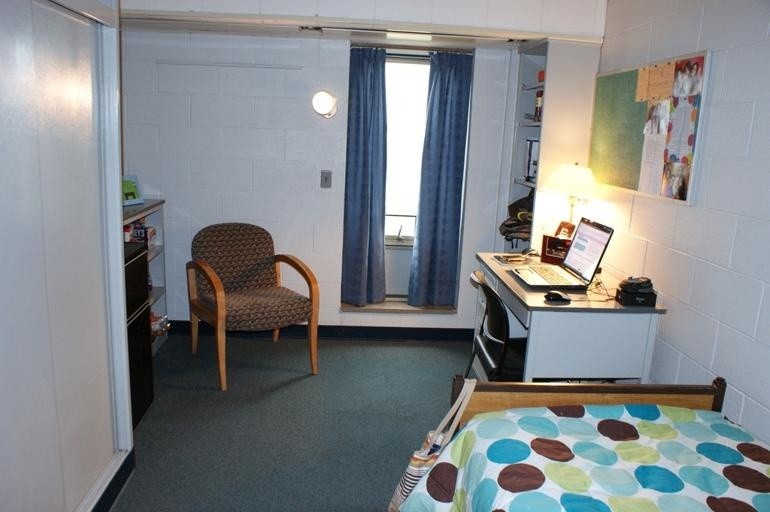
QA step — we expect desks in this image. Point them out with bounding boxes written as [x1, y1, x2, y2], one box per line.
[472, 250, 667, 382]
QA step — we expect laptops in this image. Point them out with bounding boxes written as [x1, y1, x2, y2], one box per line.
[512, 215, 614, 289]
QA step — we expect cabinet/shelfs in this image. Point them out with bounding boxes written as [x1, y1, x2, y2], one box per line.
[124, 241, 155, 431]
[503, 35, 549, 253]
[0, 0, 136, 512]
[123, 199, 172, 357]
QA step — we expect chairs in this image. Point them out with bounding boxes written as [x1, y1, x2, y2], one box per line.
[184, 221, 323, 393]
[464, 272, 528, 382]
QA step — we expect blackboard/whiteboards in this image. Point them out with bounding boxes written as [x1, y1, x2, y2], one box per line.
[589, 50, 711, 205]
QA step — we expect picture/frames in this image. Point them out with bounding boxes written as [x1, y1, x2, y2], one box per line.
[122, 174, 145, 207]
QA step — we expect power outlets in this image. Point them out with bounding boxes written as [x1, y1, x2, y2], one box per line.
[320, 169, 332, 189]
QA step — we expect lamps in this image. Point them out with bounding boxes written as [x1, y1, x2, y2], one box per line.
[311, 89, 339, 120]
[547, 160, 607, 223]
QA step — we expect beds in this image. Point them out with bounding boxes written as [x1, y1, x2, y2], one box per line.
[396, 371, 770, 512]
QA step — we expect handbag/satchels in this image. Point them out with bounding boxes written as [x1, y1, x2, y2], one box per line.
[387, 378, 478, 512]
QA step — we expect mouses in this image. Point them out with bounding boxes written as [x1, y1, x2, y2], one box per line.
[540, 288, 570, 305]
